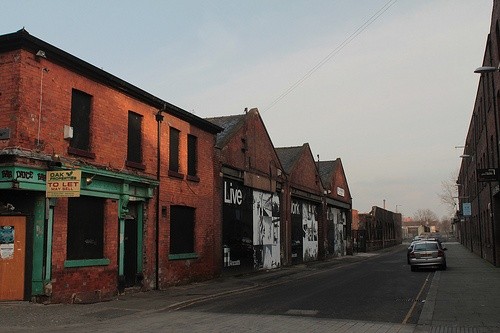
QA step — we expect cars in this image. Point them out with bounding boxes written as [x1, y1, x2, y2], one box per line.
[406, 237, 441, 264]
[410, 242, 448, 272]
